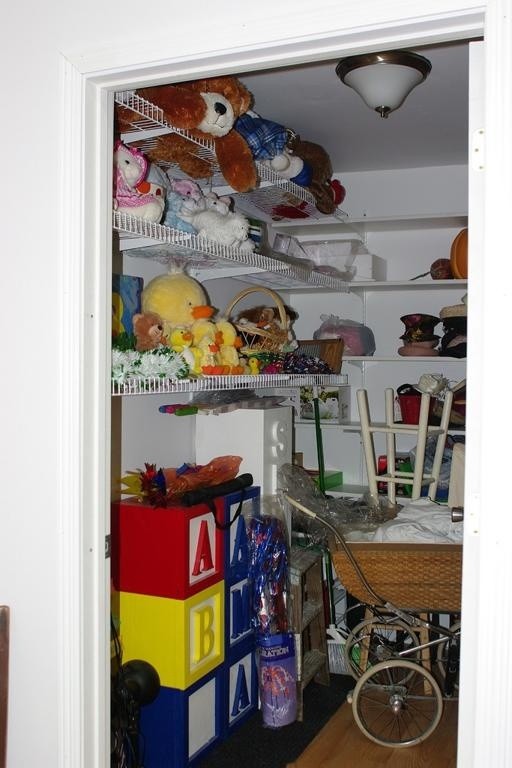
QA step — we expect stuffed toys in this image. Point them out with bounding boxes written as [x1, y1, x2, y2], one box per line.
[112, 76, 346, 374]
[111, 268, 298, 376]
[112, 77, 345, 252]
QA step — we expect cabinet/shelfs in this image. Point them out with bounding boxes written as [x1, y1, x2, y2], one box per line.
[112, 91, 351, 397]
[266, 213, 466, 508]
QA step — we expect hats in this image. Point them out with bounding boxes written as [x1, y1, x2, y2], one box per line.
[398, 313, 440, 356]
[439, 304, 468, 357]
[430, 378, 465, 428]
[411, 374, 441, 399]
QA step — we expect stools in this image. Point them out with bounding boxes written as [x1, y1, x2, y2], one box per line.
[291, 548, 331, 721]
[356, 389, 453, 507]
[357, 604, 432, 699]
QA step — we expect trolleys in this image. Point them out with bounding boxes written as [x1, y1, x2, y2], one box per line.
[276, 491, 460, 748]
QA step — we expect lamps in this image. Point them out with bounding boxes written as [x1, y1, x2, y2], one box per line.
[337, 52, 431, 120]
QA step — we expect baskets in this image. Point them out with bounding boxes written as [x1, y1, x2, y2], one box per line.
[222, 286, 289, 354]
